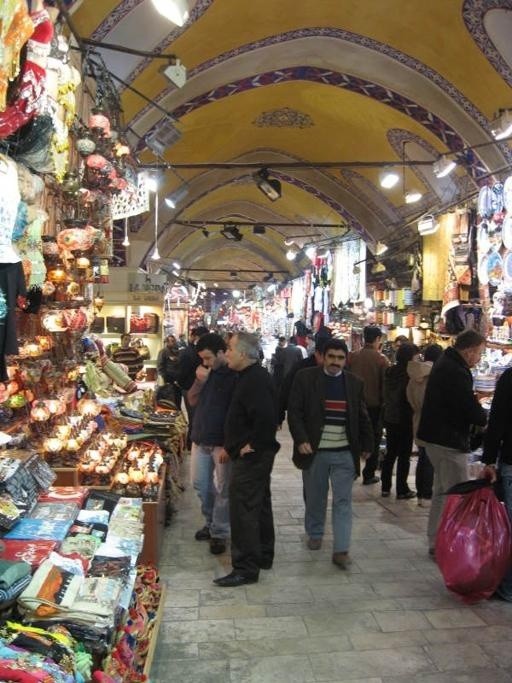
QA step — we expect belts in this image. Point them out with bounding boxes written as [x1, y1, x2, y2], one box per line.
[321, 445, 350, 453]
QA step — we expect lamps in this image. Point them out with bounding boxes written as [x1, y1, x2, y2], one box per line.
[252, 167, 282, 203]
[263, 272, 279, 279]
[135, 167, 167, 190]
[163, 260, 185, 277]
[431, 139, 510, 180]
[350, 238, 417, 276]
[123, 80, 183, 157]
[378, 162, 451, 236]
[283, 234, 350, 260]
[229, 272, 242, 278]
[200, 223, 268, 242]
[487, 110, 511, 139]
[123, 47, 187, 90]
[123, 124, 191, 209]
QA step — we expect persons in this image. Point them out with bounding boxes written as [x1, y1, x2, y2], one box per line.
[111, 333, 144, 382]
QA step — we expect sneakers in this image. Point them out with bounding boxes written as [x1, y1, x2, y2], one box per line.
[331, 550, 353, 566]
[417, 496, 432, 508]
[307, 537, 322, 550]
[382, 489, 390, 497]
[210, 536, 227, 556]
[397, 490, 417, 500]
[362, 476, 380, 485]
[194, 525, 211, 540]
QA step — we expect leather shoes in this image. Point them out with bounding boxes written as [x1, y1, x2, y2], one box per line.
[211, 570, 258, 587]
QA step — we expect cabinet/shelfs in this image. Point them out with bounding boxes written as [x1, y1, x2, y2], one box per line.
[0, 445, 166, 682]
[91, 300, 165, 386]
[15, 306, 170, 547]
[165, 302, 189, 346]
